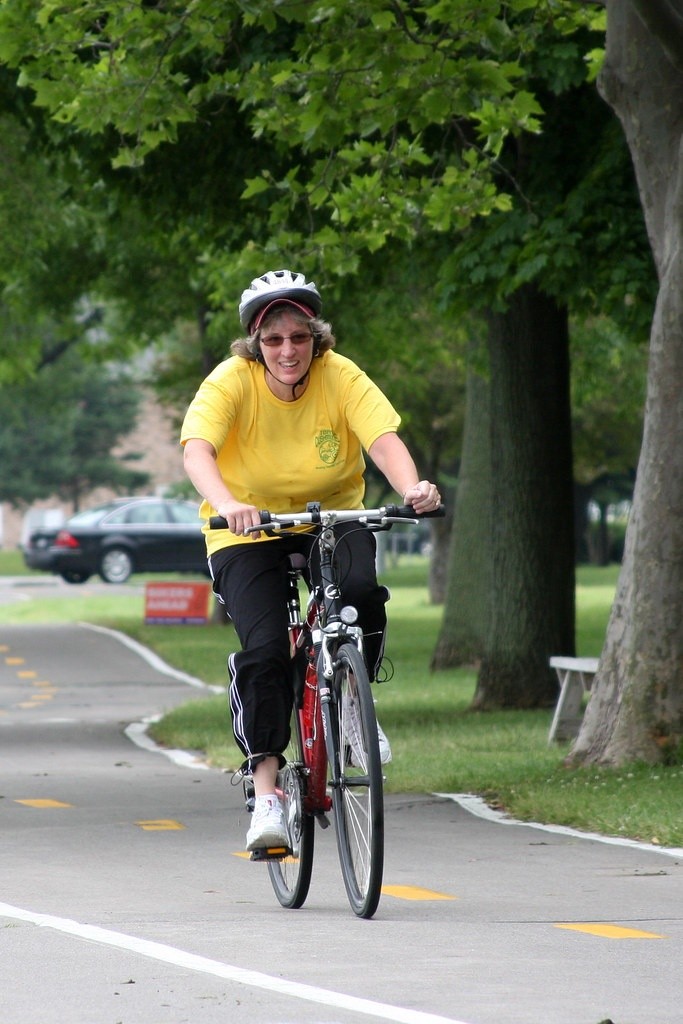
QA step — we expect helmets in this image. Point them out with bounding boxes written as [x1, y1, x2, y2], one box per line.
[239, 269, 322, 337]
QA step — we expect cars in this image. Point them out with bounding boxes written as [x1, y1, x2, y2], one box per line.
[21, 496, 209, 584]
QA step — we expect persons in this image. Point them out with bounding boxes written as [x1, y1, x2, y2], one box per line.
[179, 268, 442, 851]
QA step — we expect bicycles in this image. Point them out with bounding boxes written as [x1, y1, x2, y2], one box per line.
[207, 501, 448, 918]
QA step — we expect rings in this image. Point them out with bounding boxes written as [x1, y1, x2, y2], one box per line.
[432, 499, 436, 505]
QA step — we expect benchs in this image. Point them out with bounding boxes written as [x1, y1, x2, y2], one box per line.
[545, 656, 600, 747]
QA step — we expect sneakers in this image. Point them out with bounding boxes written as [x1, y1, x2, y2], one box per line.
[340, 697, 392, 769]
[245, 806, 290, 850]
